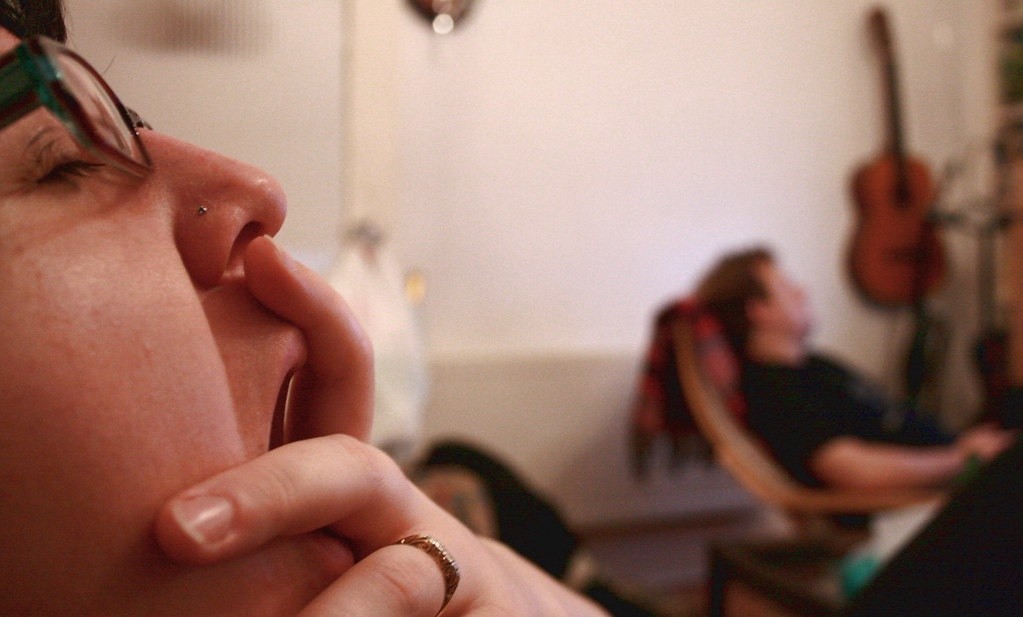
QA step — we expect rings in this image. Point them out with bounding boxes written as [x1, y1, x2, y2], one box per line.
[395, 532, 459, 617]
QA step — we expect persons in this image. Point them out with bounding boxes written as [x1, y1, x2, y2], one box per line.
[699, 248, 1023, 617]
[0, 0, 614, 617]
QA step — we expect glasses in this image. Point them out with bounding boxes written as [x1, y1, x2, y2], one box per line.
[0, 33, 154, 178]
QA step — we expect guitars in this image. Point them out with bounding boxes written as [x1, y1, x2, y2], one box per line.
[846, 3, 947, 311]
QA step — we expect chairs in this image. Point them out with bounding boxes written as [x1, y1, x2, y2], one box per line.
[661, 304, 950, 616]
